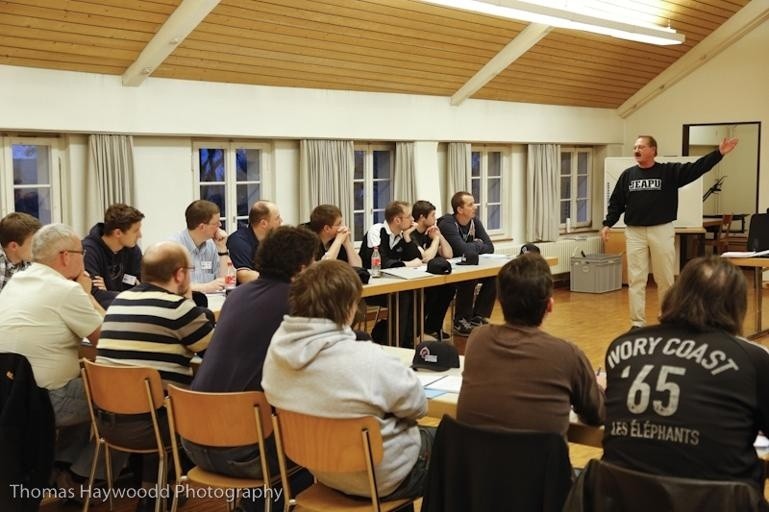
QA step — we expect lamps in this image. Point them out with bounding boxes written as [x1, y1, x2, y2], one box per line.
[423, 0, 686, 47]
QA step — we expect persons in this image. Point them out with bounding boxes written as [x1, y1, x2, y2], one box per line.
[0, 192, 769, 512]
[601, 133, 739, 333]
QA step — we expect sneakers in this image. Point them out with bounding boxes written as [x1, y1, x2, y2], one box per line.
[453, 318, 473, 337]
[418, 334, 438, 343]
[471, 314, 492, 326]
[429, 330, 451, 340]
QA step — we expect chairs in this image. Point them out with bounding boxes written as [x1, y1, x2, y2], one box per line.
[747, 213, 769, 257]
[692, 214, 733, 258]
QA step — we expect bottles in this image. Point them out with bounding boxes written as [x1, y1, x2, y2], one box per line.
[371, 246, 382, 277]
[225, 262, 237, 293]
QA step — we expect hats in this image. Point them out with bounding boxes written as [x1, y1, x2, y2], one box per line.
[426, 257, 452, 275]
[516, 244, 540, 258]
[455, 250, 479, 266]
[353, 267, 370, 284]
[412, 340, 460, 372]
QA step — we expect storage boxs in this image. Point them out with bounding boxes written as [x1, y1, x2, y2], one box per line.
[568, 255, 623, 294]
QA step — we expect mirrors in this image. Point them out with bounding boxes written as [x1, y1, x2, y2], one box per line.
[682, 121, 761, 219]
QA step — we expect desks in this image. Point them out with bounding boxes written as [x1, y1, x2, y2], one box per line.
[605, 218, 724, 288]
[75, 254, 769, 503]
[726, 258, 769, 341]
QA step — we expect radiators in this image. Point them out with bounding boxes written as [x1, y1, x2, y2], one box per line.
[494, 236, 603, 275]
